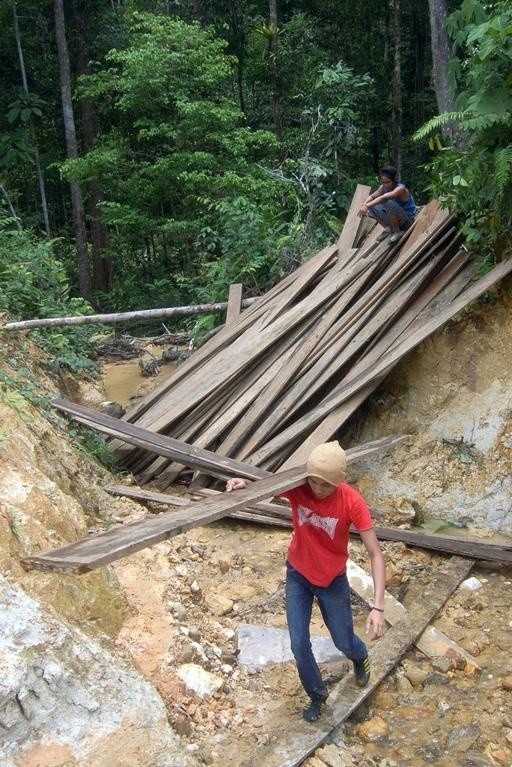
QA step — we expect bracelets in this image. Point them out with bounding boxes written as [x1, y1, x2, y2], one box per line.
[371, 606, 384, 612]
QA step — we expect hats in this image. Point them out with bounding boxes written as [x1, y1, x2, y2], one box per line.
[379, 166, 396, 181]
[306, 440, 346, 488]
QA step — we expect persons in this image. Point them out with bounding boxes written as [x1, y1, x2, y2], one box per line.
[224, 436, 387, 721]
[355, 163, 416, 245]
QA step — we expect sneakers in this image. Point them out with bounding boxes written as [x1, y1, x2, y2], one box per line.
[389, 231, 402, 242]
[303, 698, 325, 721]
[376, 231, 390, 241]
[353, 658, 370, 687]
